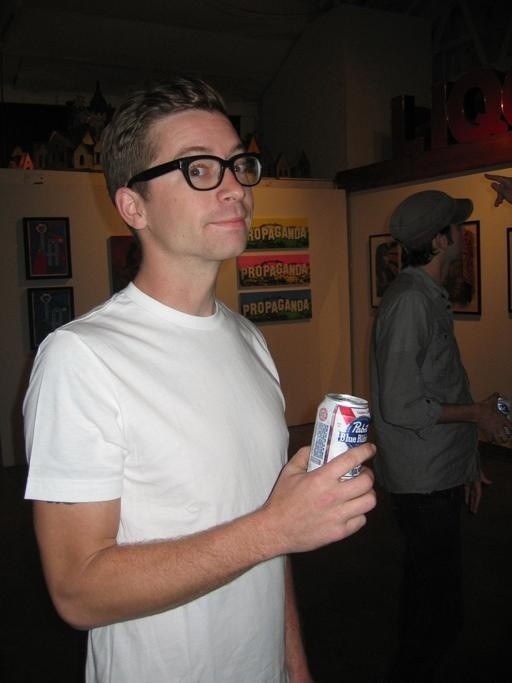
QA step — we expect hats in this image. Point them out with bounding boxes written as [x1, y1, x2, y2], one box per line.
[387, 191, 473, 253]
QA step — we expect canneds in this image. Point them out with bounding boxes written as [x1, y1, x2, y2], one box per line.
[496, 396, 512, 436]
[306, 393, 371, 481]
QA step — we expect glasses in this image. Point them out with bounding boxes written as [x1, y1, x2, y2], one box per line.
[128, 153, 262, 192]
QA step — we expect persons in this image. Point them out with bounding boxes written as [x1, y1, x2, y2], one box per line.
[21, 80, 378, 682]
[484, 173, 512, 207]
[369, 191, 510, 514]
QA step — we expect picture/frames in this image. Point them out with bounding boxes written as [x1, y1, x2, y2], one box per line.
[23, 216, 74, 350]
[369, 219, 480, 315]
